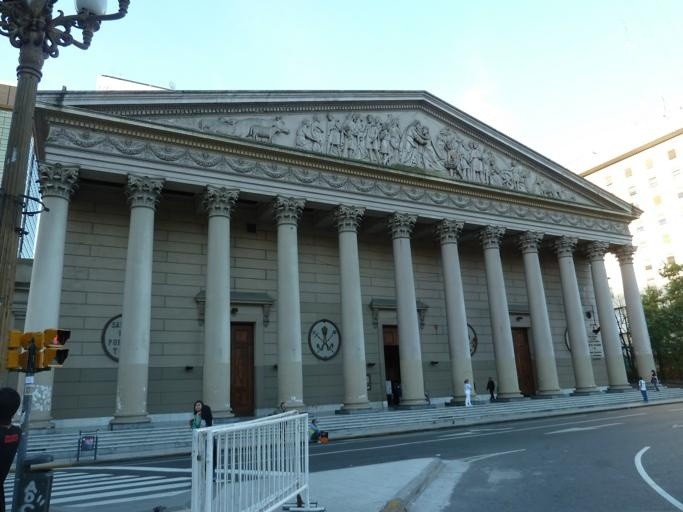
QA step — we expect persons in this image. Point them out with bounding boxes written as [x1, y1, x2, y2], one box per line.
[308, 420, 323, 443]
[295, 113, 519, 192]
[651, 370, 659, 391]
[200, 406, 217, 482]
[275, 402, 286, 414]
[487, 377, 496, 401]
[639, 377, 648, 401]
[0, 388, 22, 512]
[464, 379, 473, 406]
[191, 401, 203, 429]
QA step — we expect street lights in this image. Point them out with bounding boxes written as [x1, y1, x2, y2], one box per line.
[0, 1, 128, 387]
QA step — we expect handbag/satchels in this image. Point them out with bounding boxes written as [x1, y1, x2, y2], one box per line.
[320, 437, 329, 443]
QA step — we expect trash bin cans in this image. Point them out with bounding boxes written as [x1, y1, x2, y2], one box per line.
[11, 453, 54, 512]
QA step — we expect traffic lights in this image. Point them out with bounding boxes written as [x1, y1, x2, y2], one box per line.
[7, 328, 71, 374]
